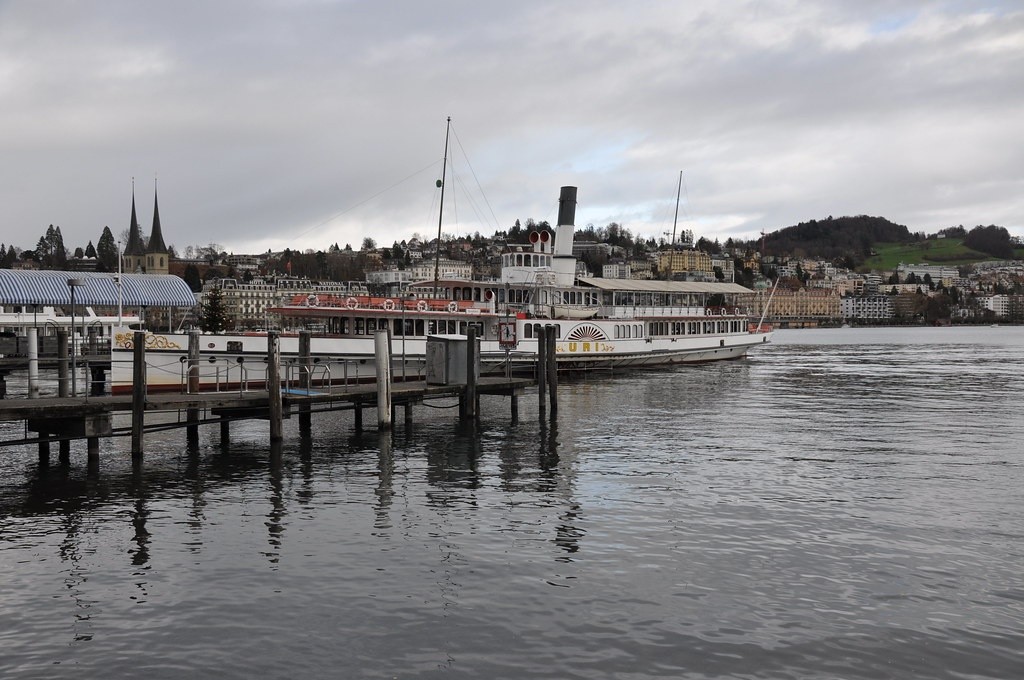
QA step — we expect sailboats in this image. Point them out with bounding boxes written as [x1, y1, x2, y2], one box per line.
[113, 115, 769, 365]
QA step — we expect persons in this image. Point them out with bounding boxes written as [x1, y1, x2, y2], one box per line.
[526, 310, 531, 319]
[622, 296, 633, 304]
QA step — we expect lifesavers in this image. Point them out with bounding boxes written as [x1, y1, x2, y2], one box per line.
[707, 308, 712, 317]
[721, 307, 726, 315]
[447, 301, 458, 313]
[746, 307, 751, 315]
[346, 297, 359, 309]
[734, 308, 740, 315]
[417, 300, 429, 313]
[305, 295, 320, 307]
[383, 300, 395, 312]
